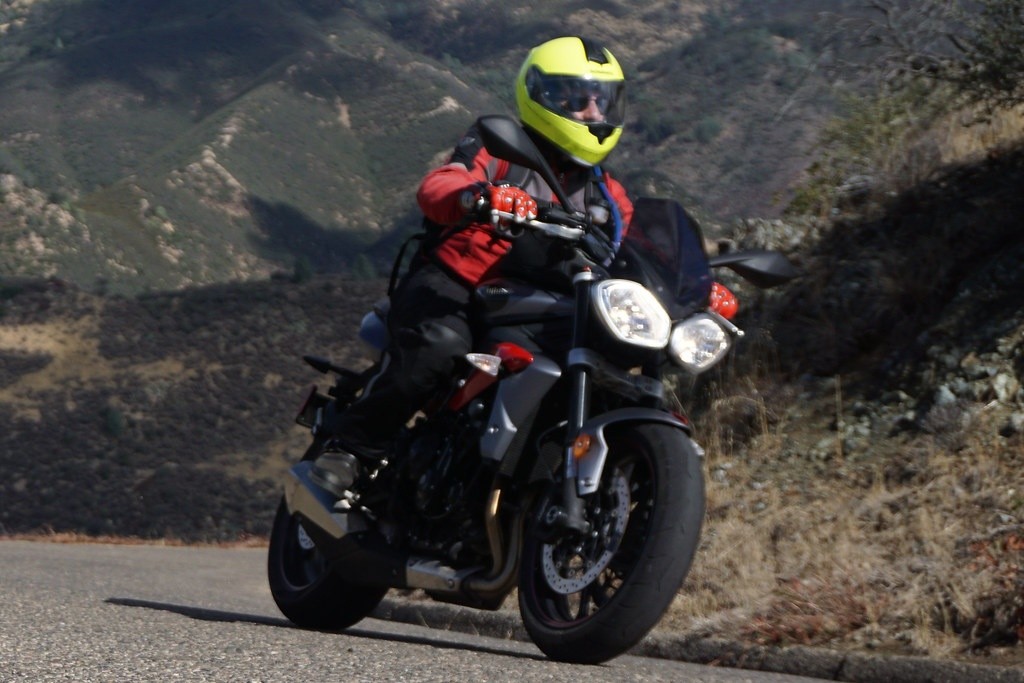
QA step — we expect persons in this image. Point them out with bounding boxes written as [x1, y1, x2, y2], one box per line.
[307, 37, 739, 558]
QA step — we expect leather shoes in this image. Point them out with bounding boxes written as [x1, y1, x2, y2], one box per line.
[309, 435, 360, 499]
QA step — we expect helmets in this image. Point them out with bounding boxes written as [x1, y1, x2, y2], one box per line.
[514, 36, 630, 166]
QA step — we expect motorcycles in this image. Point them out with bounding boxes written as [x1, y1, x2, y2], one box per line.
[265, 114, 798, 668]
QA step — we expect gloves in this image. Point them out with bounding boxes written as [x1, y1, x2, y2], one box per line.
[708, 282, 738, 319]
[462, 180, 539, 231]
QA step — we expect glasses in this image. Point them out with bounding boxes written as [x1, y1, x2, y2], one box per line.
[562, 96, 613, 113]
